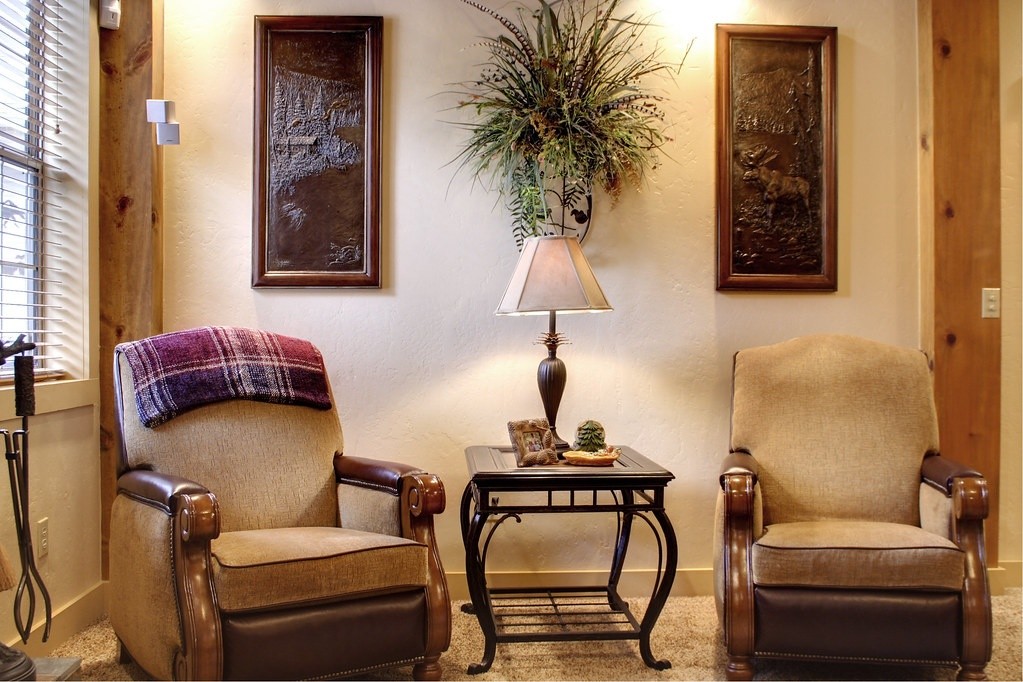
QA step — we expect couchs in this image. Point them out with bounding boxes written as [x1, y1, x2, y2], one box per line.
[107, 325, 453, 682]
[711, 332, 994, 682]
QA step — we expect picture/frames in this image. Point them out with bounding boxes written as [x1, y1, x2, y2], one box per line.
[714, 22, 838, 294]
[250, 14, 383, 291]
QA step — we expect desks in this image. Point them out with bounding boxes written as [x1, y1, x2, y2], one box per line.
[459, 445, 678, 676]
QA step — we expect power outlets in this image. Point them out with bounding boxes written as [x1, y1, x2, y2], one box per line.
[37, 517, 50, 558]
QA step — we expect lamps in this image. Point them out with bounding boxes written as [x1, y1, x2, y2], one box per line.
[495, 233, 617, 461]
[0, 540, 36, 682]
